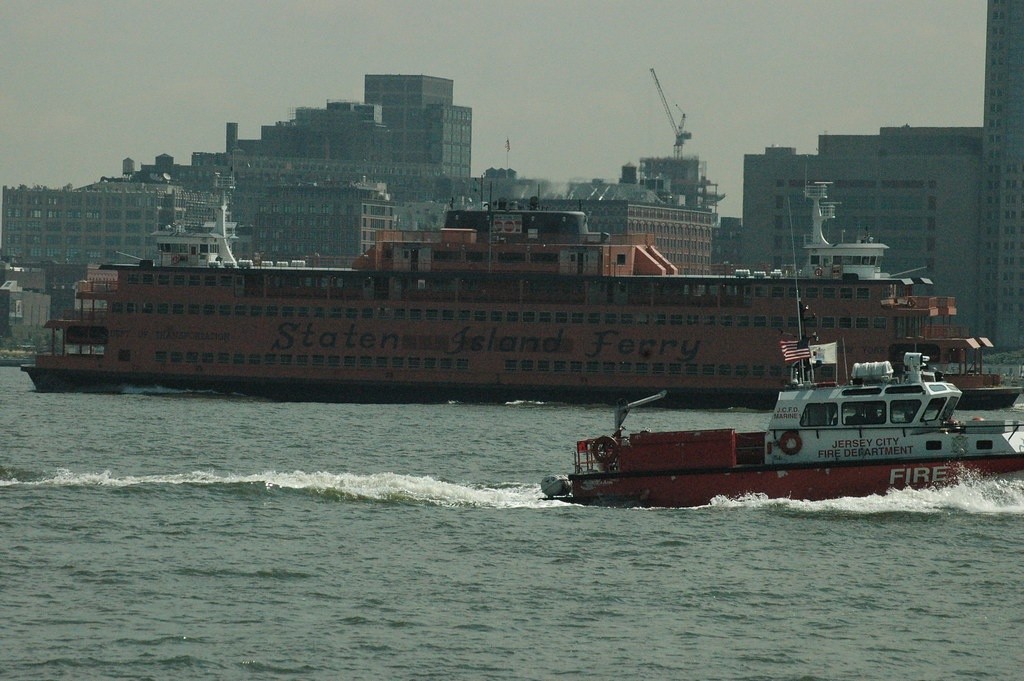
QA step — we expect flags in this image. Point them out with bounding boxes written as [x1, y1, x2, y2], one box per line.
[809, 342, 836, 365]
[780, 340, 810, 362]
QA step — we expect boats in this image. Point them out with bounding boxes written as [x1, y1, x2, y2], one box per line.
[539, 193, 1024, 508]
[20, 173, 1024, 411]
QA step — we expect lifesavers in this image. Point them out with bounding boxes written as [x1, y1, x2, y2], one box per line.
[780, 431, 803, 454]
[593, 435, 618, 464]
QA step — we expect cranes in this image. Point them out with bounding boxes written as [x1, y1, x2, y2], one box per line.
[649, 68, 692, 158]
